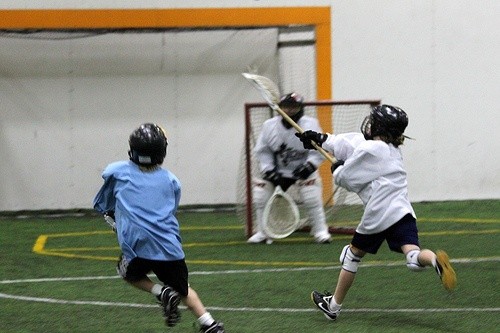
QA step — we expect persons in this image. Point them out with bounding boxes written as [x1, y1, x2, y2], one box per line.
[93, 122, 226, 333]
[296, 104, 456, 321]
[246, 94, 334, 243]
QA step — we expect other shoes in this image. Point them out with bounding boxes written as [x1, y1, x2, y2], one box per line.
[314, 231, 333, 244]
[247, 231, 267, 244]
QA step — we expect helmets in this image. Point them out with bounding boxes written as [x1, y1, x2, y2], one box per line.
[128, 123, 167, 169]
[277, 92, 303, 129]
[361, 105, 408, 148]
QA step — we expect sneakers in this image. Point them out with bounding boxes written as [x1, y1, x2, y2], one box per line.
[311, 290, 342, 321]
[156, 285, 181, 327]
[435, 250, 457, 289]
[200, 321, 225, 333]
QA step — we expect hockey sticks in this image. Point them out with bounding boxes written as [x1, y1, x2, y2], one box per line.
[261, 184, 300, 239]
[240, 72, 336, 164]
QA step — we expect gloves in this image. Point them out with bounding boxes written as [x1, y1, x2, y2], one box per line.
[104, 210, 117, 229]
[295, 130, 328, 150]
[293, 162, 315, 179]
[264, 170, 281, 185]
[330, 160, 344, 173]
